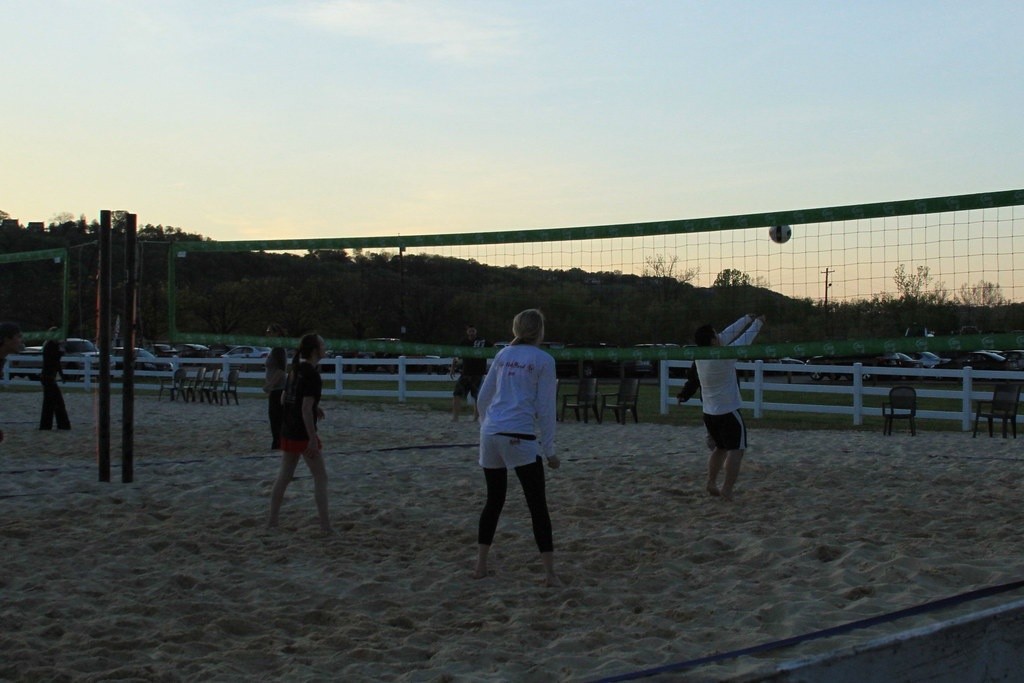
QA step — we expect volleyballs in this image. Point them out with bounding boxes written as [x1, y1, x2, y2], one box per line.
[769, 224, 791, 244]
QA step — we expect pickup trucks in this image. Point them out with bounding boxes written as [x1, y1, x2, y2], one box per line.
[25, 339, 116, 382]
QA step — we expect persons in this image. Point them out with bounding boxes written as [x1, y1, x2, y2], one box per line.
[266, 332, 330, 529]
[450, 324, 487, 422]
[677, 312, 768, 501]
[38, 327, 72, 430]
[473, 309, 561, 586]
[0, 321, 25, 370]
[262, 346, 289, 449]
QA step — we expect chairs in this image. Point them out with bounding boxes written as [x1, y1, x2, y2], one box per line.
[175, 367, 209, 403]
[211, 370, 239, 406]
[192, 368, 222, 404]
[600, 377, 639, 426]
[881, 385, 918, 436]
[562, 376, 598, 424]
[156, 368, 186, 402]
[972, 383, 1023, 438]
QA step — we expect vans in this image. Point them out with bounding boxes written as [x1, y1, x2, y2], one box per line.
[629, 342, 683, 377]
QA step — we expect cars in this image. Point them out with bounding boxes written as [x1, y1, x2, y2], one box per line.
[174, 344, 214, 360]
[549, 342, 636, 378]
[109, 346, 172, 373]
[997, 349, 1024, 372]
[148, 342, 183, 359]
[222, 346, 273, 360]
[877, 353, 945, 381]
[930, 351, 1006, 379]
[205, 342, 240, 357]
[807, 354, 874, 383]
